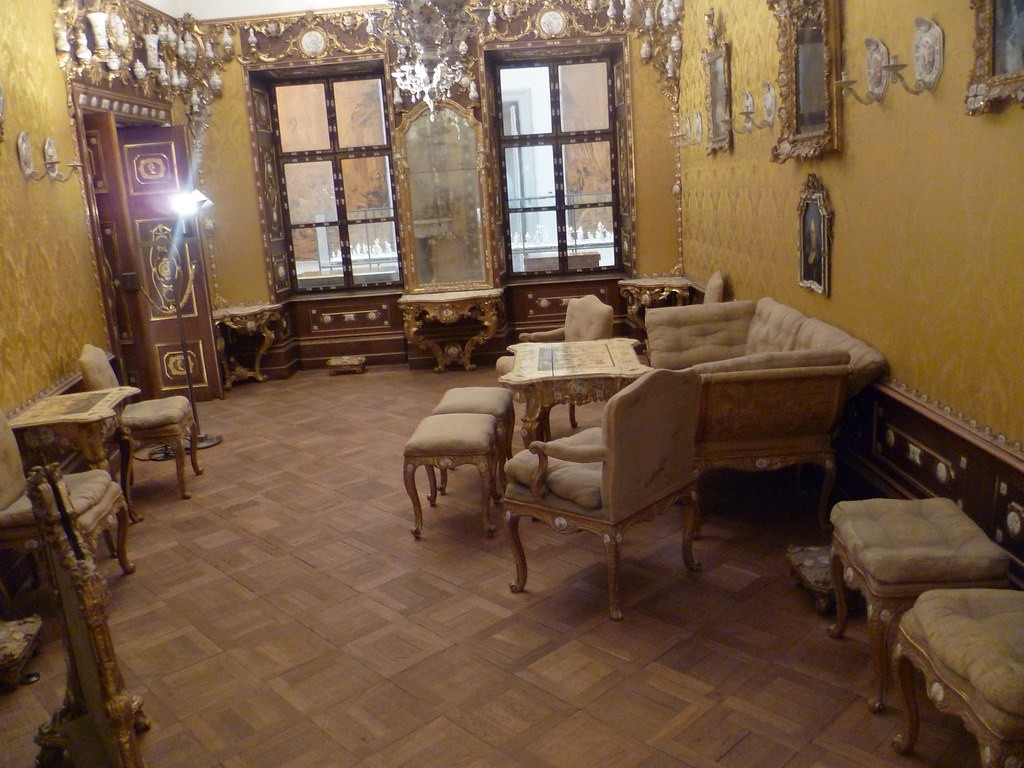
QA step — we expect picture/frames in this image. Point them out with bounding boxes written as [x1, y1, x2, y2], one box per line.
[962, 0, 1024, 118]
[796, 173, 835, 298]
[765, 0, 843, 164]
[700, 7, 733, 158]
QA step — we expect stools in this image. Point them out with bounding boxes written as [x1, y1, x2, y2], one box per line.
[827, 497, 1011, 716]
[890, 587, 1024, 768]
[402, 413, 503, 537]
[432, 386, 517, 494]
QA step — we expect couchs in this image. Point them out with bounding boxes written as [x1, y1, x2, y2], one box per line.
[642, 297, 887, 536]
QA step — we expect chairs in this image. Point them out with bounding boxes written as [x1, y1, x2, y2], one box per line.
[496, 294, 613, 427]
[79, 343, 205, 501]
[1, 409, 135, 614]
[704, 270, 724, 303]
[501, 367, 704, 621]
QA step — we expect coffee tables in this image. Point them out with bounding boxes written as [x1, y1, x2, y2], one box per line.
[498, 338, 654, 522]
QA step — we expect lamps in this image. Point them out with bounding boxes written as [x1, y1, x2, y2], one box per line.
[363, 0, 492, 123]
[167, 197, 223, 449]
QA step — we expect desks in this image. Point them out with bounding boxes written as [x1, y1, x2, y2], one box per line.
[212, 303, 284, 391]
[10, 386, 146, 576]
[396, 287, 505, 374]
[618, 277, 692, 353]
